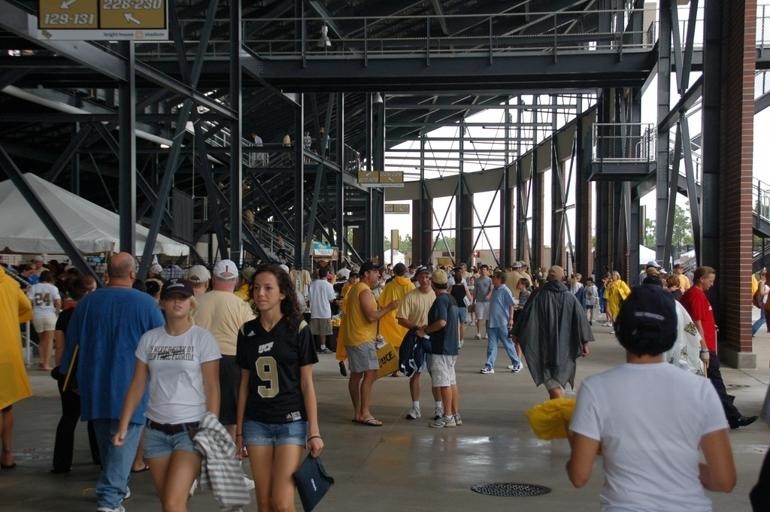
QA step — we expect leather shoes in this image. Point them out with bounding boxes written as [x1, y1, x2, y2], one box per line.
[729, 415, 758, 431]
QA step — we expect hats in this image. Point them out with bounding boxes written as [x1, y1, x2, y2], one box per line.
[431, 268, 449, 285]
[187, 264, 210, 283]
[616, 284, 679, 338]
[547, 266, 566, 283]
[360, 261, 380, 274]
[645, 260, 683, 276]
[212, 259, 239, 280]
[510, 261, 522, 269]
[158, 277, 195, 301]
[415, 265, 431, 278]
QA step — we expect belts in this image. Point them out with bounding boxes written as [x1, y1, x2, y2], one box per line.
[145, 419, 202, 437]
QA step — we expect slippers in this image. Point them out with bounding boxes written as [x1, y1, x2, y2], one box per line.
[132, 462, 155, 473]
[350, 417, 385, 426]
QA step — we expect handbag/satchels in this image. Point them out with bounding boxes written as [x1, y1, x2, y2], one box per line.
[289, 454, 338, 512]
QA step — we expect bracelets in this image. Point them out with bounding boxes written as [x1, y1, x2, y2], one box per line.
[236, 433, 242, 437]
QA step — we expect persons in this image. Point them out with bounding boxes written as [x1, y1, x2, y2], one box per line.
[473, 260, 769, 374]
[566, 284, 736, 508]
[0, 252, 324, 511]
[310, 259, 472, 428]
[507, 264, 594, 401]
[680, 266, 759, 430]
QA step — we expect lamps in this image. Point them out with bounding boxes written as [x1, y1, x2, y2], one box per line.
[315, 15, 333, 48]
[373, 92, 383, 104]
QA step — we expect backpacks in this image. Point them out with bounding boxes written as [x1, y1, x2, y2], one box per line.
[752, 282, 764, 310]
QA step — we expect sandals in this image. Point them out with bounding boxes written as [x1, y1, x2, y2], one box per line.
[1, 460, 18, 470]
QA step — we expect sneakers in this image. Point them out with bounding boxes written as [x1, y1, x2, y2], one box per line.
[511, 363, 522, 373]
[426, 402, 471, 430]
[319, 348, 335, 354]
[480, 366, 496, 375]
[96, 484, 135, 512]
[405, 409, 423, 421]
